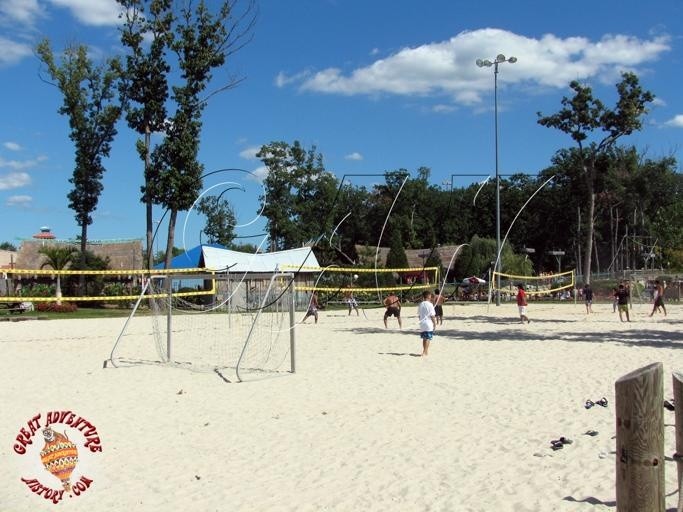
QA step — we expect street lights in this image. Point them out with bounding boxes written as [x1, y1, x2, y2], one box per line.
[475, 53, 517, 307]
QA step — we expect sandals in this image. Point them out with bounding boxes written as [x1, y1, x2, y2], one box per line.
[552, 437, 572, 450]
[585, 397, 608, 409]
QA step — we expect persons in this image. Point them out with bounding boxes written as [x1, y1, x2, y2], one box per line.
[434, 289, 448, 324]
[526, 270, 583, 301]
[344, 284, 359, 316]
[461, 286, 496, 303]
[418, 290, 437, 357]
[648, 279, 677, 317]
[301, 290, 321, 323]
[612, 280, 632, 322]
[383, 290, 403, 330]
[583, 285, 593, 314]
[517, 282, 532, 324]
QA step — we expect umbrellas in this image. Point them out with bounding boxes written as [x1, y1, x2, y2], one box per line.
[463, 275, 486, 292]
